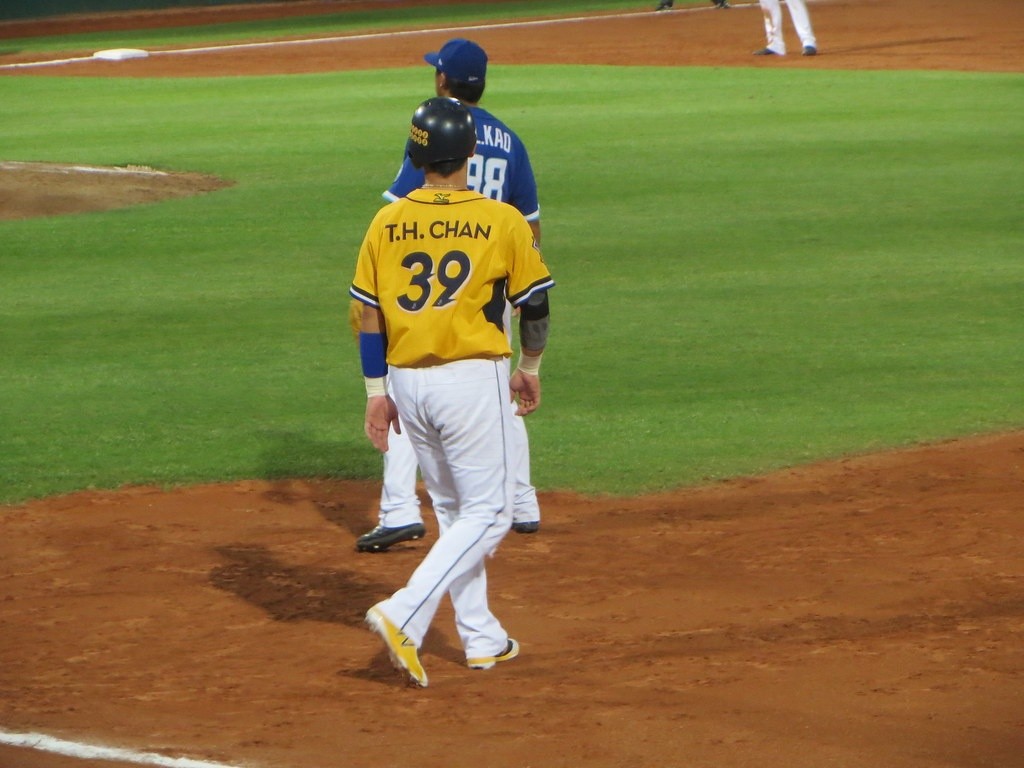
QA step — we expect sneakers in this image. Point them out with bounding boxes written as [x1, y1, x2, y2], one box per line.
[366, 604, 428, 689]
[510, 521, 539, 534]
[355, 523, 427, 552]
[468, 638, 519, 671]
[753, 48, 773, 55]
[802, 46, 817, 55]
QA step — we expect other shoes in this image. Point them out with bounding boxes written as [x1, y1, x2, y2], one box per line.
[656, 5, 669, 10]
[720, 2, 730, 9]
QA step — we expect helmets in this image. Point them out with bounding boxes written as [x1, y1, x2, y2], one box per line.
[408, 97, 477, 167]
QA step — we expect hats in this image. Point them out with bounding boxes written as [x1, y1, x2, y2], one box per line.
[426, 38, 487, 82]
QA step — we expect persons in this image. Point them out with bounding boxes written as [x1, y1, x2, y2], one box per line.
[349, 96, 557, 690]
[355, 36, 543, 553]
[657, 0, 818, 58]
